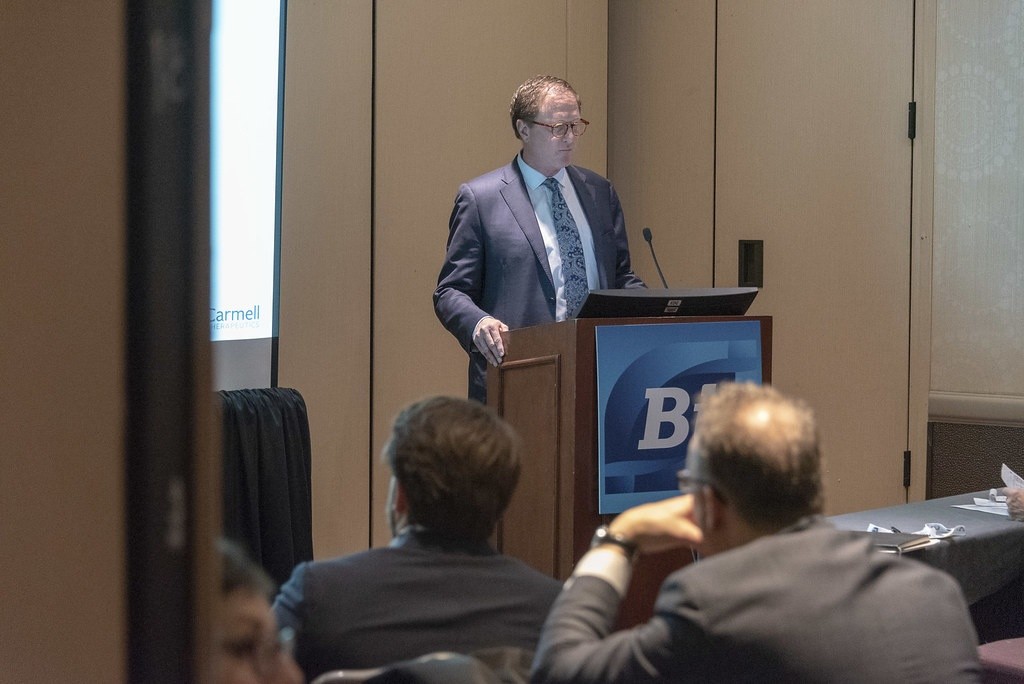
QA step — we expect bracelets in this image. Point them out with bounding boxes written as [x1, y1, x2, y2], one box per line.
[591, 526, 639, 555]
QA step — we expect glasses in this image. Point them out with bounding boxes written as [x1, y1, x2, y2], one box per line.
[519, 119, 590, 138]
[676, 470, 724, 505]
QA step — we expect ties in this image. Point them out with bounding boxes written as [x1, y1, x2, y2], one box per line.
[542, 178, 588, 319]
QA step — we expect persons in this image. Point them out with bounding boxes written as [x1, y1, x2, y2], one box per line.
[275, 394, 562, 684]
[532, 379, 986, 684]
[201, 535, 306, 684]
[433, 74, 649, 405]
[1002, 485, 1024, 522]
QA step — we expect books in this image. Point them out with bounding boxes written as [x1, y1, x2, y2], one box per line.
[848, 530, 929, 552]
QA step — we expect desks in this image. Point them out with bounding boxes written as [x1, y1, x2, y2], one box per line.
[817, 486, 1024, 632]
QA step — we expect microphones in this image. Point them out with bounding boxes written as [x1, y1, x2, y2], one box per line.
[642, 227, 672, 289]
